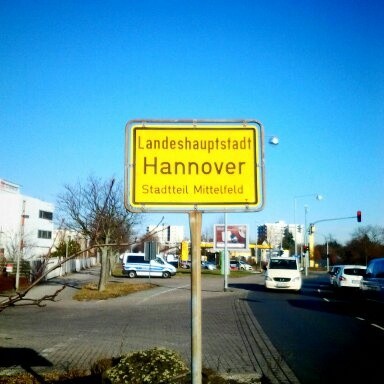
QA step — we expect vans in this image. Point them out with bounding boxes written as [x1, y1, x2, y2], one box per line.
[122, 253, 176, 279]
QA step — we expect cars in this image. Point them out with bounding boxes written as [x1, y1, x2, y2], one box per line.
[264, 256, 304, 291]
[329, 258, 384, 300]
[201, 260, 253, 272]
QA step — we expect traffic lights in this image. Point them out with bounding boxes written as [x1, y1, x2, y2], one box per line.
[303, 245, 307, 253]
[357, 211, 362, 222]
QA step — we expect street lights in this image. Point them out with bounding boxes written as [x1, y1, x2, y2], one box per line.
[304, 194, 322, 278]
